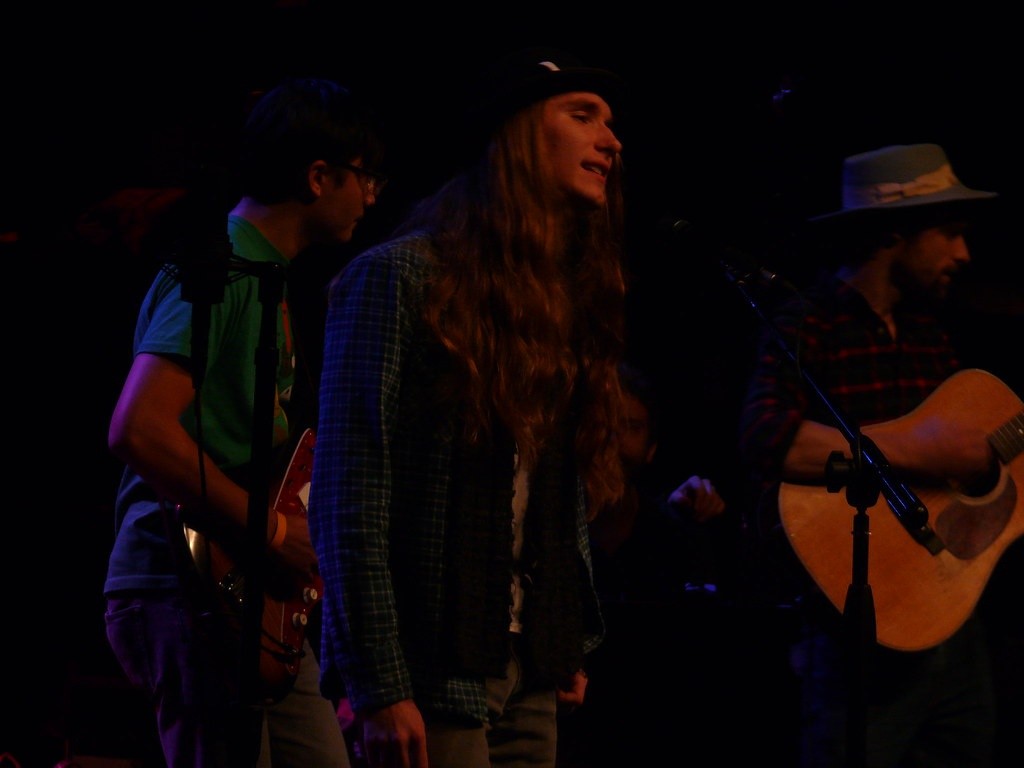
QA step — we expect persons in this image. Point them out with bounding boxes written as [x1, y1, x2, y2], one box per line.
[558, 142, 996, 768]
[310, 61, 625, 768]
[105, 67, 378, 768]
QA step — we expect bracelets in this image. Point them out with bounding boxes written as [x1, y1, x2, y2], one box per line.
[270, 512, 288, 550]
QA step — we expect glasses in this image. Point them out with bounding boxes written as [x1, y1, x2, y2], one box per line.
[329, 160, 387, 195]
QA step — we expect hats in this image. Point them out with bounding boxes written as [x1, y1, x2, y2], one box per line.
[805, 142, 999, 221]
[467, 44, 621, 155]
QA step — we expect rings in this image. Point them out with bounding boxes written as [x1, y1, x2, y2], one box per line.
[367, 751, 386, 761]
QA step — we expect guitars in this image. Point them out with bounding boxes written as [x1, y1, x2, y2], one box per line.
[777, 367, 1023, 652]
[173, 426, 324, 707]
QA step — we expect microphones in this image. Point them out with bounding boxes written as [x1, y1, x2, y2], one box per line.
[653, 215, 718, 249]
